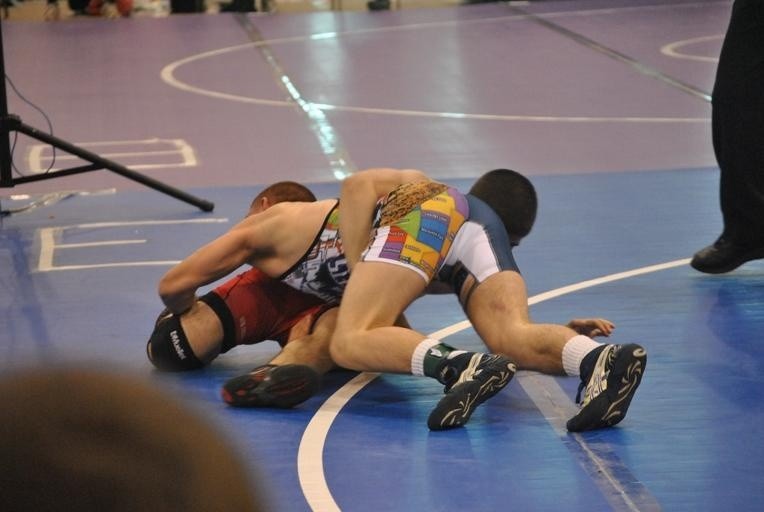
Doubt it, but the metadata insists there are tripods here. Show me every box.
[0,0,215,213]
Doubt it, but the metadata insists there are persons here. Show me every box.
[691,0,764,274]
[157,182,648,431]
[148,169,615,410]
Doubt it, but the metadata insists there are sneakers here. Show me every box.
[691,230,763,275]
[427,352,517,431]
[566,343,647,432]
[221,363,318,408]
